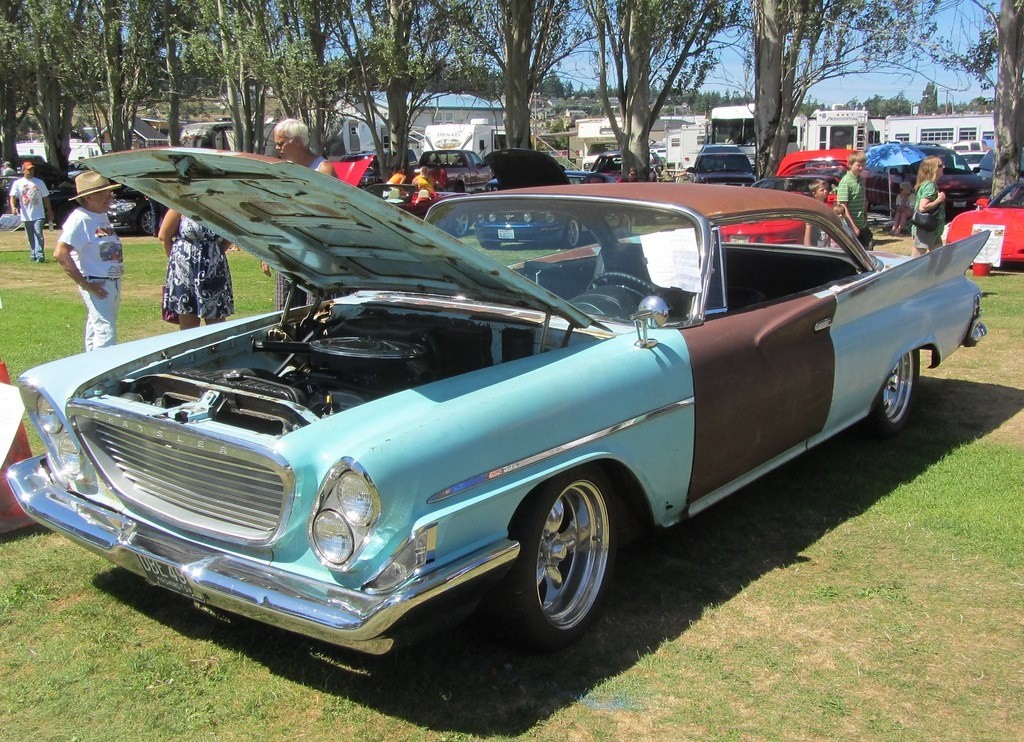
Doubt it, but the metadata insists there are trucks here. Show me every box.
[704,104,807,175]
[421,119,506,161]
[180,122,279,161]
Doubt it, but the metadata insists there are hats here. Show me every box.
[899,182,912,194]
[68,171,122,201]
[2,162,11,167]
[22,162,36,170]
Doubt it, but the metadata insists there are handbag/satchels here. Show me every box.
[909,207,941,231]
[160,285,179,325]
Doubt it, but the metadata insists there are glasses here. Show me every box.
[94,191,114,197]
[938,163,944,168]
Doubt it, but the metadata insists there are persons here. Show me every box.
[0,161,13,187]
[890,181,917,237]
[410,165,437,192]
[835,150,872,250]
[8,163,52,264]
[384,167,406,191]
[49,173,131,350]
[261,118,342,313]
[803,178,827,249]
[821,204,846,252]
[910,155,948,259]
[155,204,234,332]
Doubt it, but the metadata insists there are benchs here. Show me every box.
[525,242,857,322]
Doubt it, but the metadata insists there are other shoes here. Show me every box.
[30,254,45,263]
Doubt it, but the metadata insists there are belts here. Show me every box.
[83,275,120,281]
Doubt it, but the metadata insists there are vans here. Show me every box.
[340,148,418,191]
[360,184,474,237]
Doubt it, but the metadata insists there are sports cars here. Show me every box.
[945,182,1024,265]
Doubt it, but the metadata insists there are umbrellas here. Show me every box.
[862,141,929,237]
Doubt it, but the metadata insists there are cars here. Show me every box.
[686,152,756,188]
[472,148,632,249]
[866,145,993,223]
[951,139,1024,210]
[4,144,994,667]
[1,153,168,238]
[649,150,663,173]
[885,140,902,145]
[694,144,743,154]
[583,150,658,184]
[412,150,493,195]
[715,149,869,247]
